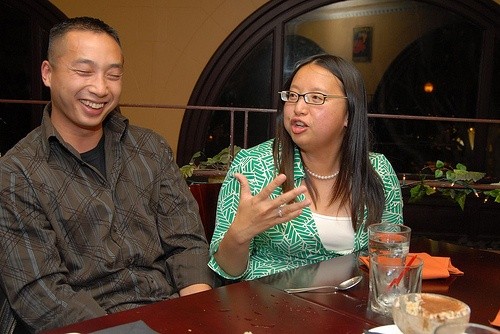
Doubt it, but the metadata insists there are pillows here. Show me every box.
[359,251,464,280]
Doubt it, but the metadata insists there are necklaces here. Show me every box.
[305,167,339,180]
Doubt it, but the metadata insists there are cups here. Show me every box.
[367,223,424,325]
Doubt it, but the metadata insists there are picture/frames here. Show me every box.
[352,24,373,62]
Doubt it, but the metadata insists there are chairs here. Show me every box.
[190,182,221,240]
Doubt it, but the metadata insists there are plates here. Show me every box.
[362,324,403,334]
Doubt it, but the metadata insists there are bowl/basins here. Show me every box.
[433,322,500,334]
[393,293,471,334]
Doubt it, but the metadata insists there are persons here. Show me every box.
[0,17,216,334]
[207,52,404,289]
[403,127,464,173]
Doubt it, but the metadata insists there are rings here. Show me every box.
[279,207,283,217]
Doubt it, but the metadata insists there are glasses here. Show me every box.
[277,91,348,106]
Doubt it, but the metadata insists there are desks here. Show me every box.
[36,279,376,334]
[256,236,500,331]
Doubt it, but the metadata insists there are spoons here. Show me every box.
[283,275,363,293]
